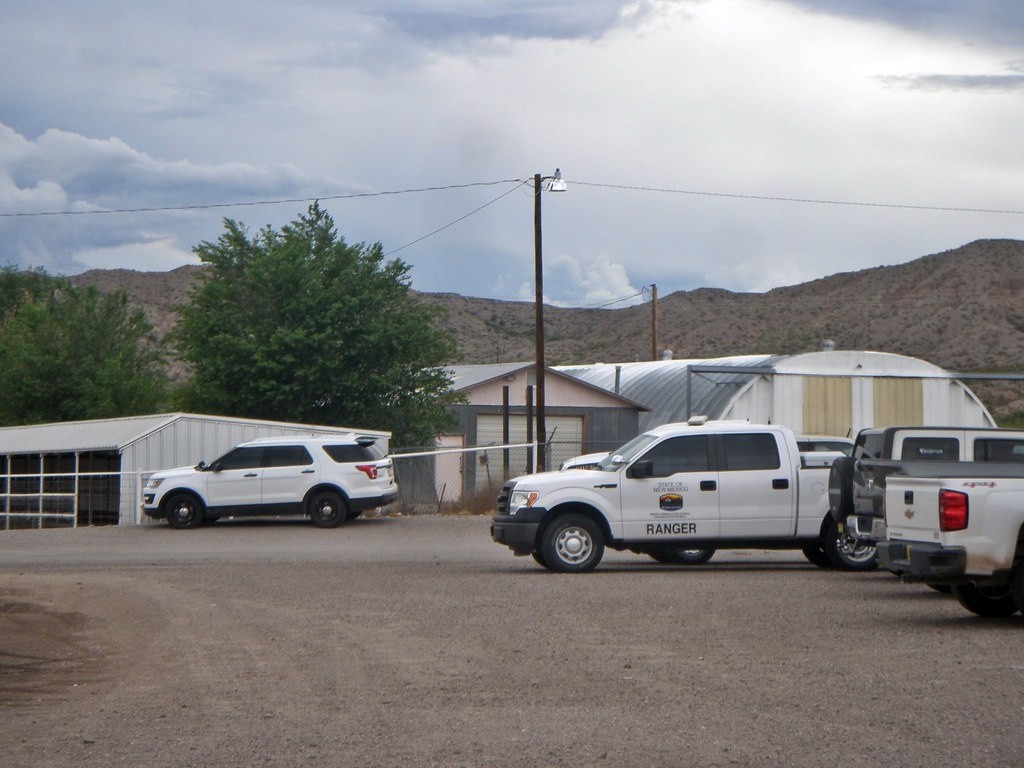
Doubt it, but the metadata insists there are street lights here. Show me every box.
[533,166,567,468]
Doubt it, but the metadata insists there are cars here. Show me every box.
[561,428,878,565]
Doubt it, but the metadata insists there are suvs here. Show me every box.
[839,423,1024,590]
[141,432,400,529]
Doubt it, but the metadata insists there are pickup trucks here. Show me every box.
[488,417,884,574]
[874,475,1024,623]
